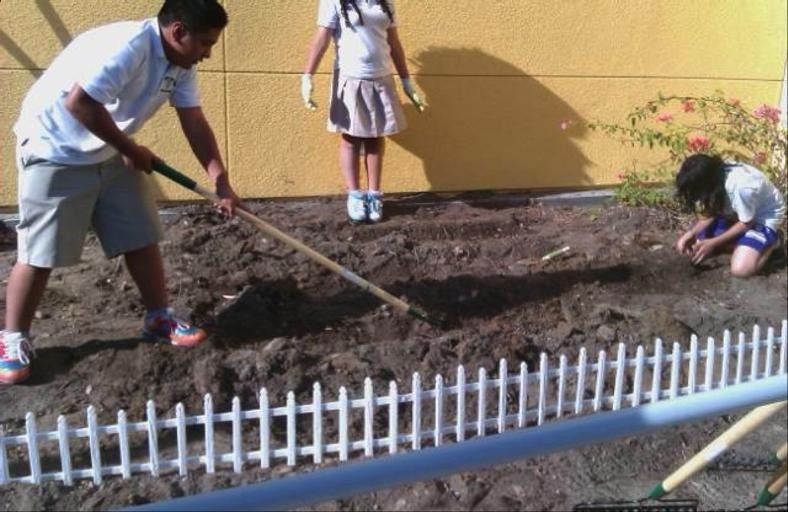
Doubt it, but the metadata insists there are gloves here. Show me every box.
[301,72,317,112]
[402,78,430,113]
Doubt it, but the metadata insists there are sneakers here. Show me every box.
[0,328,38,386]
[346,189,367,222]
[366,190,385,223]
[137,305,209,349]
[769,228,786,266]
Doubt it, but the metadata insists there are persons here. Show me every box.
[0,0,240,384]
[673,153,788,279]
[301,0,424,224]
